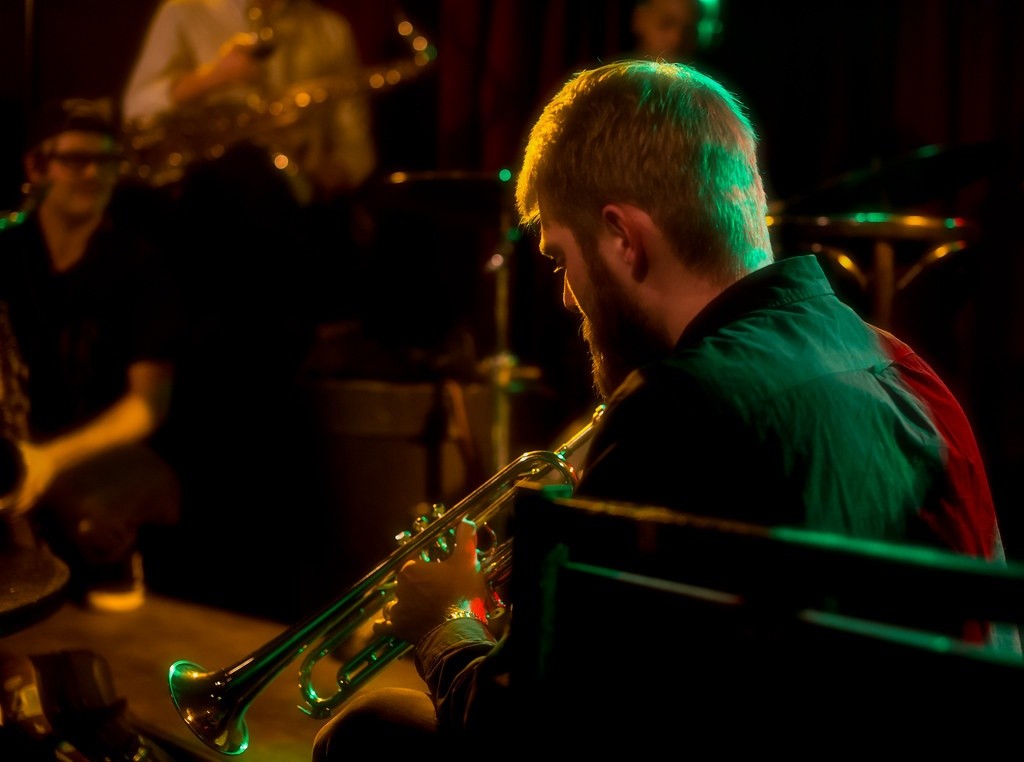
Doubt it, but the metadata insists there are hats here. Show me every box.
[26,97,119,144]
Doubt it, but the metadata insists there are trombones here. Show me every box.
[168,398,609,760]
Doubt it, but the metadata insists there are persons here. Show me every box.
[0,98,175,597]
[311,59,1023,762]
[117,3,376,616]
[628,1,723,65]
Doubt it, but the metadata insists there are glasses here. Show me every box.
[50,151,124,169]
